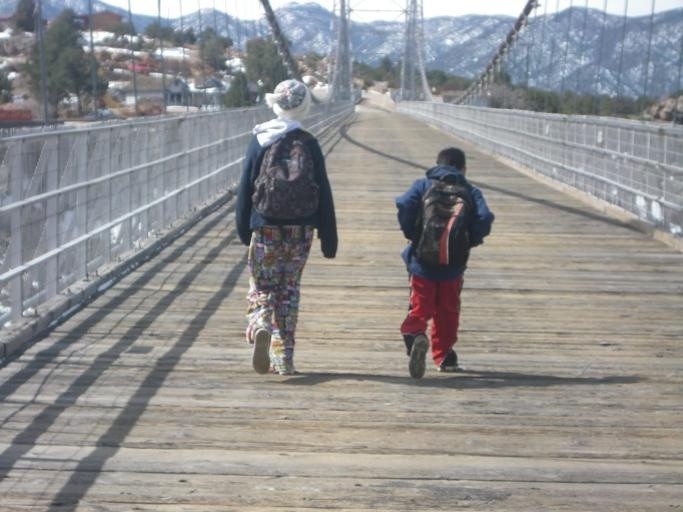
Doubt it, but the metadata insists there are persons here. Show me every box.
[396,148,495,380]
[235,80,338,376]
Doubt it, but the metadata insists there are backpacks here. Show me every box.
[414,178,475,273]
[252,131,320,220]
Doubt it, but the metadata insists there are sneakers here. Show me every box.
[252,329,271,374]
[437,351,458,372]
[273,363,295,375]
[408,334,427,378]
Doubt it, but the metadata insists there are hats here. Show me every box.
[264,78,311,121]
